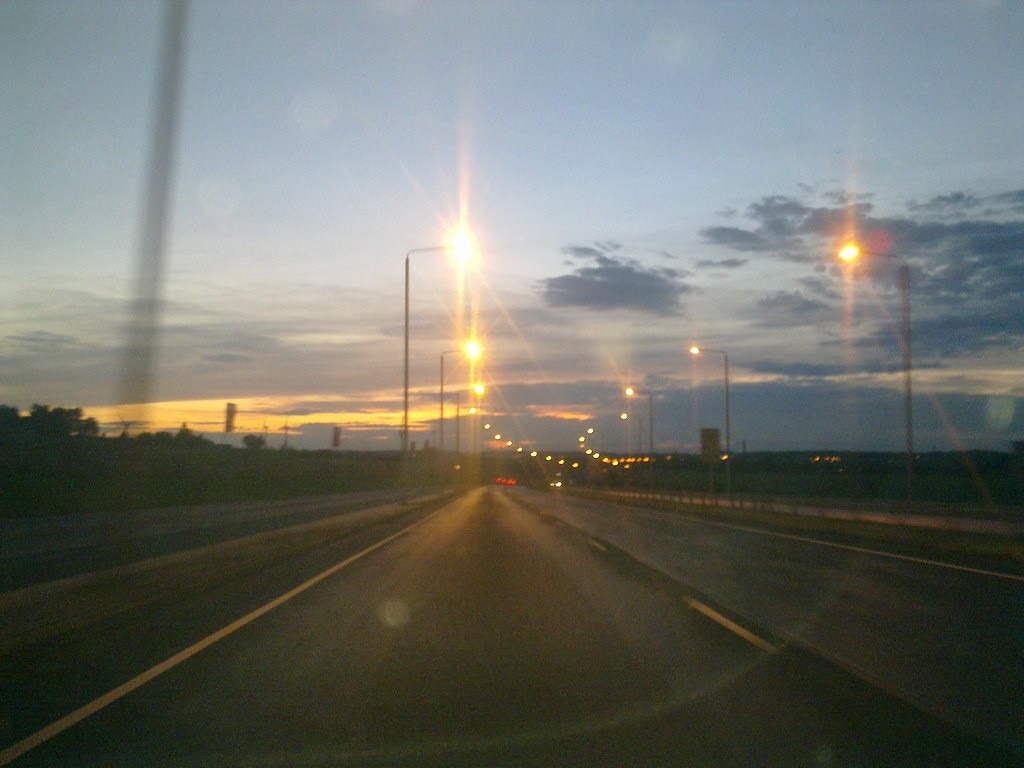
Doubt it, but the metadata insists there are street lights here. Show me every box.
[438,344,483,458]
[456,384,485,458]
[839,241,921,498]
[626,387,656,488]
[403,244,476,475]
[688,345,735,496]
